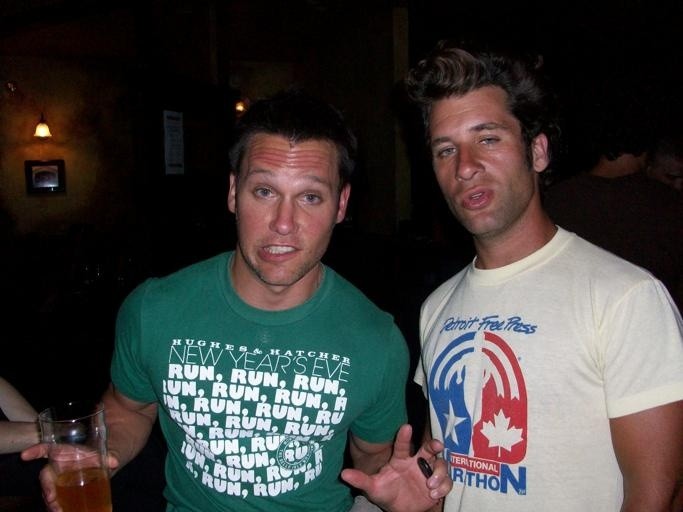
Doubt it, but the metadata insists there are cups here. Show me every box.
[38,401,111,512]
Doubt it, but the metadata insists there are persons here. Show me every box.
[0,377,41,454]
[21,91,453,512]
[405,48,682,512]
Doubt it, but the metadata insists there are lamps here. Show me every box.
[33,111,53,140]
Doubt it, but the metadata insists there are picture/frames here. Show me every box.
[24,158,66,196]
